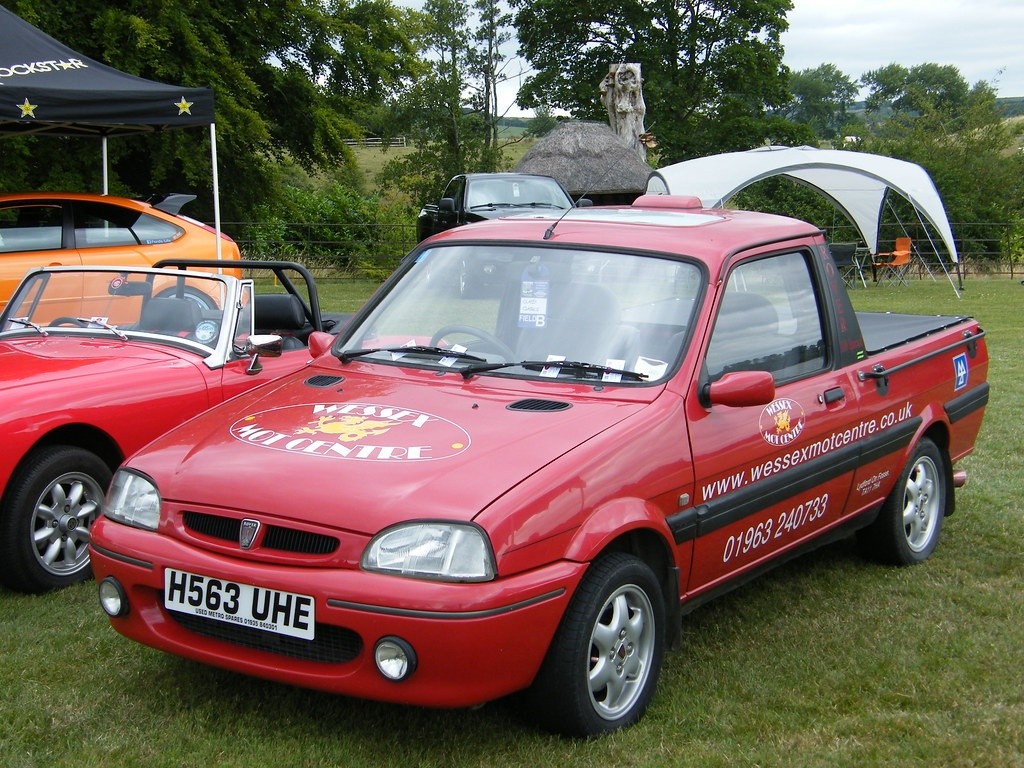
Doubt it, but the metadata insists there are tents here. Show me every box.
[0,6,226,313]
[644,146,965,292]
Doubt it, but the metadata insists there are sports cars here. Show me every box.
[0,257,448,595]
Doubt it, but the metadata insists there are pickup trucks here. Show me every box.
[88,190,992,743]
[417,172,593,296]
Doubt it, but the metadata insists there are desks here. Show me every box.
[856,248,870,288]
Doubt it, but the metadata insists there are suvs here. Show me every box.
[2,192,252,337]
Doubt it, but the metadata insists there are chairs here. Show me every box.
[873,237,911,288]
[236,294,309,355]
[828,244,857,290]
[141,296,203,338]
[703,290,778,370]
[515,281,617,364]
